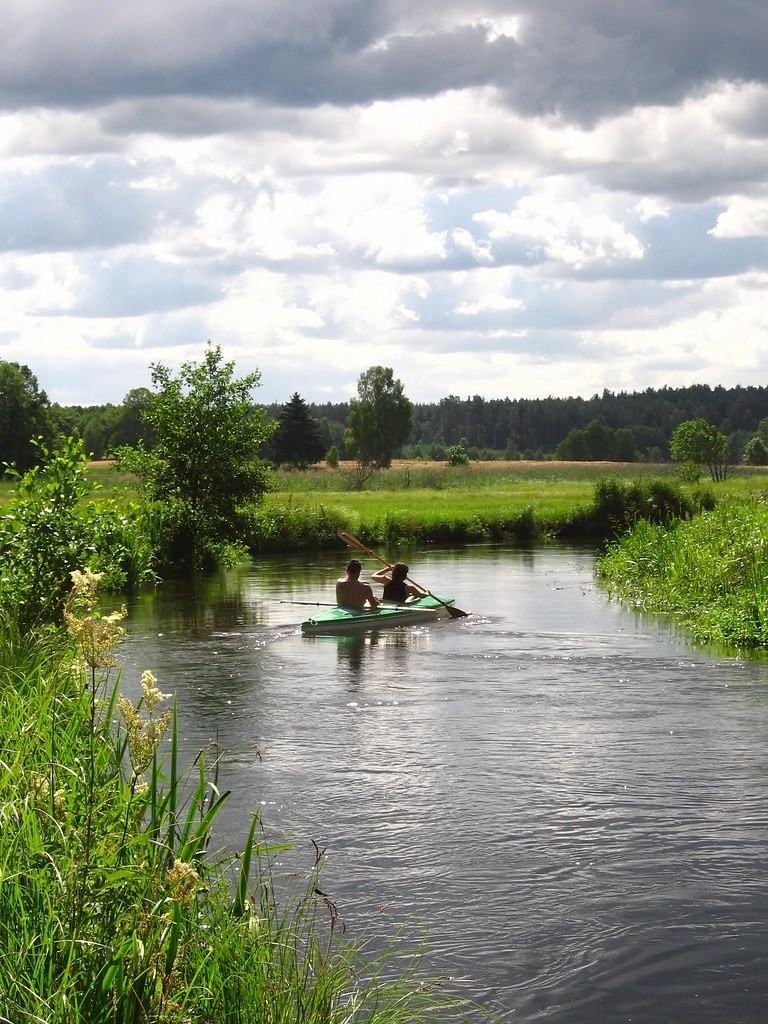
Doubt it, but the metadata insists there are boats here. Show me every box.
[299,595,456,637]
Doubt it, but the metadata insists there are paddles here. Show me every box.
[334,525,471,619]
[278,597,441,618]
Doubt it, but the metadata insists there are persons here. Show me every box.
[336,560,379,609]
[371,562,430,602]
